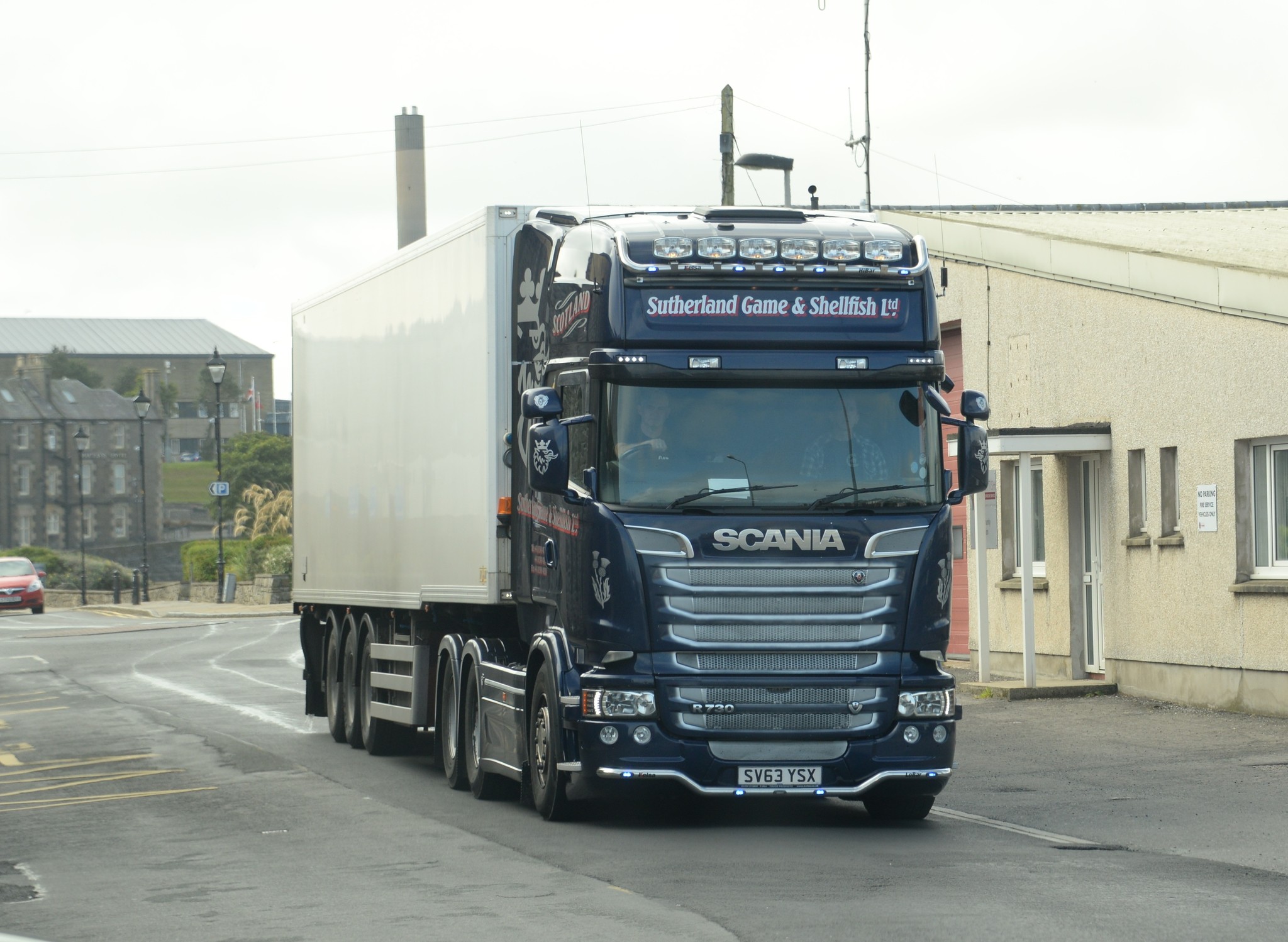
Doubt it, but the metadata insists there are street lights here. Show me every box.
[730,151,794,203]
[206,346,228,605]
[131,386,152,603]
[73,425,94,605]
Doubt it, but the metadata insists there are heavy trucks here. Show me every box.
[290,203,990,824]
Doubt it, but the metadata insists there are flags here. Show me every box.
[255,398,263,409]
[245,388,253,401]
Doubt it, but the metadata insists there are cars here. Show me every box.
[0,557,47,614]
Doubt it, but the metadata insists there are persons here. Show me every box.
[611,388,704,485]
[799,391,888,483]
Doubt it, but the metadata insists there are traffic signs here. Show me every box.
[207,482,229,497]
